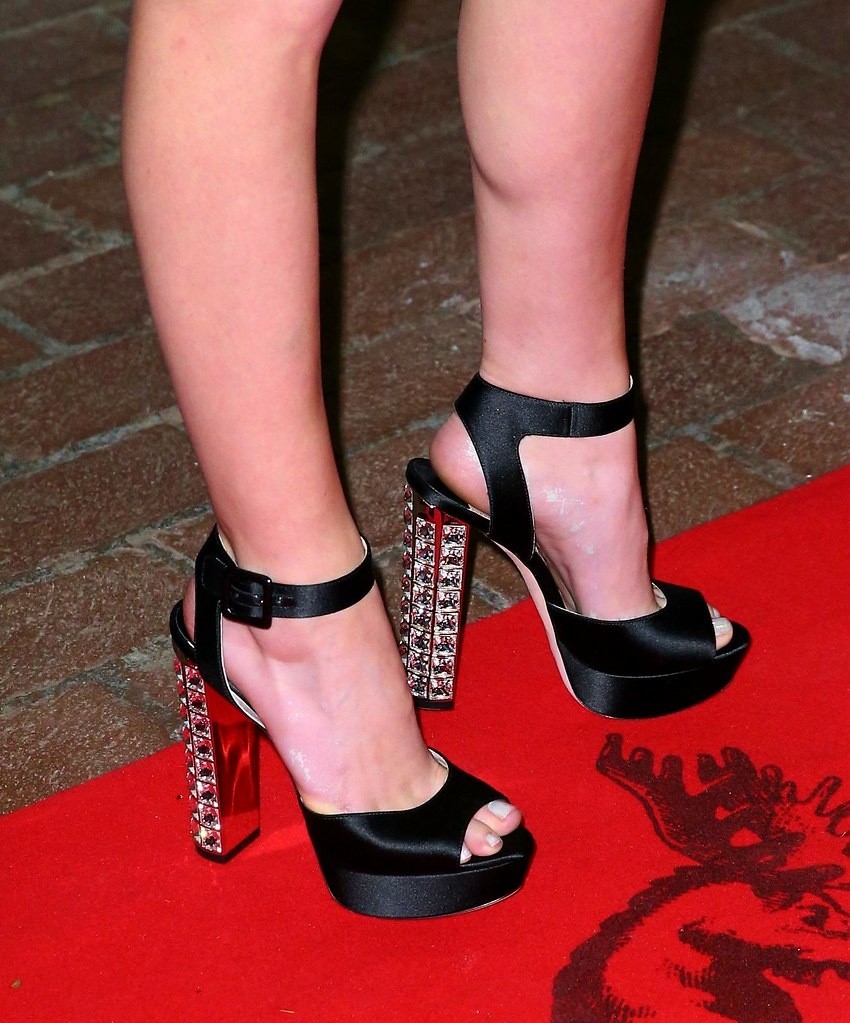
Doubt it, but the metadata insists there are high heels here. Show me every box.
[399,371,750,720]
[169,522,535,918]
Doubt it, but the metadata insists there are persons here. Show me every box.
[121,0,753,918]
